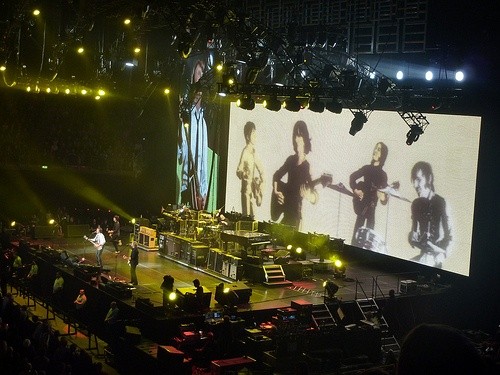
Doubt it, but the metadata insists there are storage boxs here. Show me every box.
[400,279,418,293]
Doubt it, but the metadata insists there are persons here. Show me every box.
[88,228,106,266]
[193,279,203,300]
[350,142,388,249]
[105,302,120,325]
[407,161,454,269]
[273,120,318,232]
[108,215,121,254]
[0,291,104,375]
[27,260,38,300]
[13,254,22,278]
[236,121,263,220]
[180,207,193,225]
[123,241,139,285]
[220,214,233,251]
[74,290,87,327]
[221,316,233,333]
[52,271,64,314]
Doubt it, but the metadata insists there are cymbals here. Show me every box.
[327,179,356,199]
[376,184,411,204]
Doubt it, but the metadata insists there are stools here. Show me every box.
[261,249,275,261]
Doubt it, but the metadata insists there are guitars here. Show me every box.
[350,180,400,215]
[123,254,134,267]
[105,228,113,238]
[83,235,103,250]
[270,173,333,221]
[249,174,264,208]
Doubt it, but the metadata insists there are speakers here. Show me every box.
[32,226,56,239]
[215,282,253,306]
[176,286,212,308]
[65,224,90,238]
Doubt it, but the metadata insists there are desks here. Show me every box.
[220,230,270,259]
[309,258,333,263]
[212,356,256,375]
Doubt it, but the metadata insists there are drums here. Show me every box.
[355,226,387,255]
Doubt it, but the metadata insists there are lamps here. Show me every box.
[286,245,307,260]
[0,10,464,145]
[322,281,339,300]
